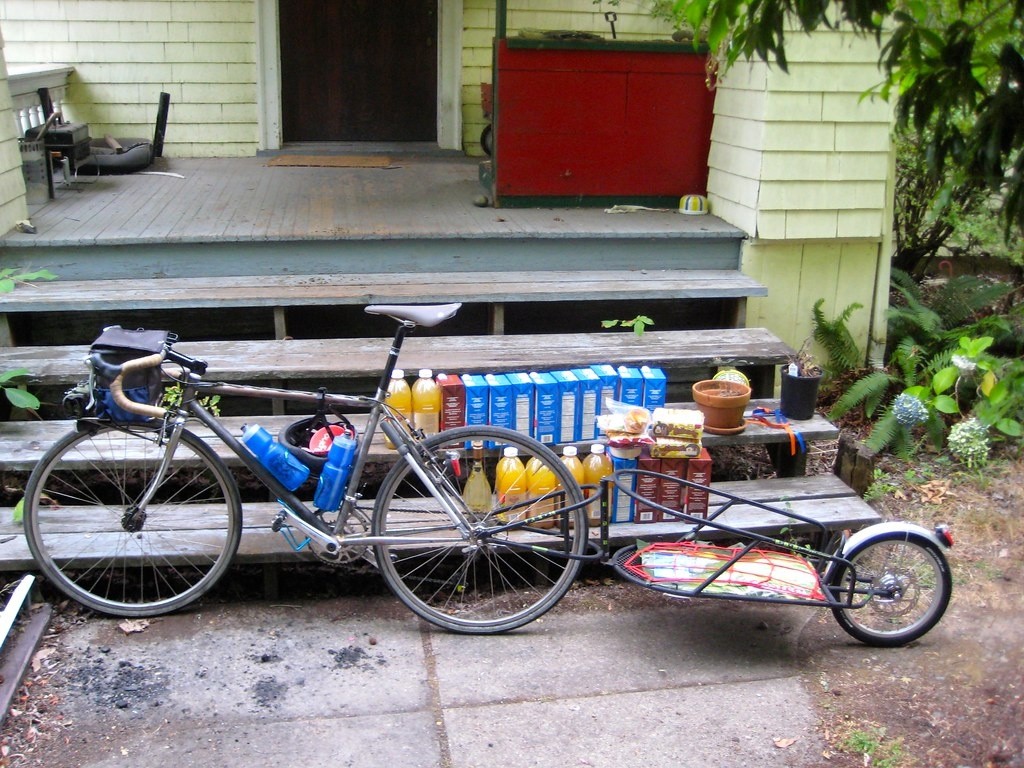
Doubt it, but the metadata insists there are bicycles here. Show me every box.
[22,303,588,637]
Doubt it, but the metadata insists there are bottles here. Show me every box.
[240,423,310,492]
[582,444,612,526]
[464,441,491,519]
[527,456,556,529]
[411,368,441,440]
[556,446,584,528]
[384,369,411,449]
[495,447,527,522]
[316,431,357,511]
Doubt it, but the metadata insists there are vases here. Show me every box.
[780,364,823,420]
[692,380,752,427]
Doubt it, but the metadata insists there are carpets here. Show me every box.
[264,154,392,169]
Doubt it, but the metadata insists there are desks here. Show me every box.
[491,34,716,210]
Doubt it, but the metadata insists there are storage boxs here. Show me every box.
[640,367,666,430]
[682,448,712,523]
[591,365,620,436]
[658,457,683,521]
[617,367,642,408]
[633,447,658,524]
[529,372,557,446]
[435,373,464,448]
[506,373,534,438]
[88,325,167,421]
[571,369,600,441]
[461,374,487,449]
[606,447,637,523]
[484,374,509,449]
[550,370,578,443]
